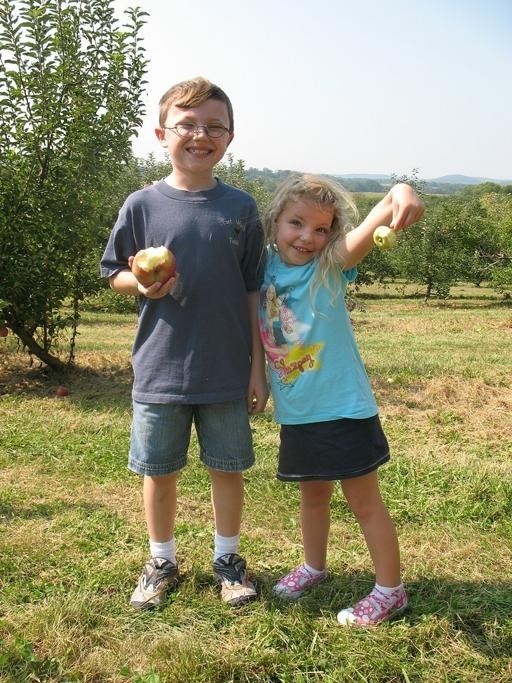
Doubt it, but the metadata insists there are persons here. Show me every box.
[100,75,270,611]
[256,172,425,629]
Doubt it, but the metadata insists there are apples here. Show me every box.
[373,226,397,248]
[57,385,69,396]
[0,327,8,337]
[131,246,177,288]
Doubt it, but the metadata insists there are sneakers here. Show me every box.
[272,565,322,601]
[334,587,407,626]
[210,554,256,603]
[126,558,177,604]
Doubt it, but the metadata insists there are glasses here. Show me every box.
[163,117,234,139]
